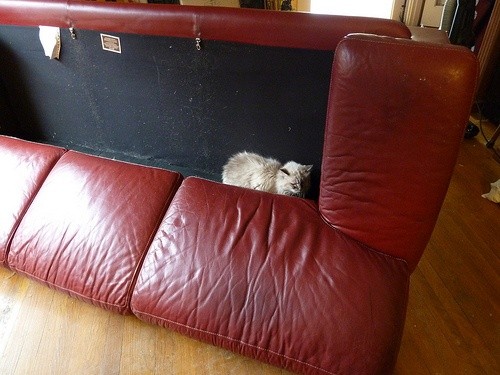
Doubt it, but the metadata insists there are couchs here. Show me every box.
[0,0,480,374]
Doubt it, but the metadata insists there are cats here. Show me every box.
[222,150,314,198]
